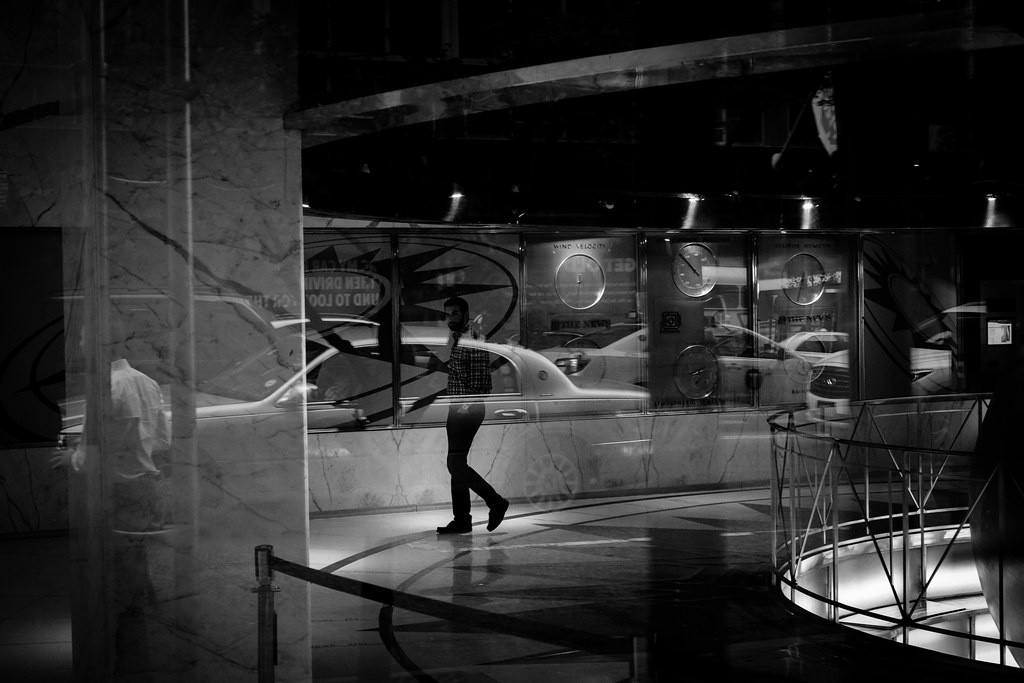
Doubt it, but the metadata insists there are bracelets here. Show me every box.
[463,404,468,410]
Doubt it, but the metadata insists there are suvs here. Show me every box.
[267,311,381,332]
[64,289,276,416]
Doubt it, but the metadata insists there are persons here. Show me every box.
[427,297,509,534]
[45,308,171,621]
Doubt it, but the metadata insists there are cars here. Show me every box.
[58,326,650,513]
[502,330,583,362]
[804,301,987,433]
[777,328,853,361]
[541,318,811,408]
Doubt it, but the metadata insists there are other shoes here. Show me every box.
[487,499,509,531]
[437,521,472,534]
